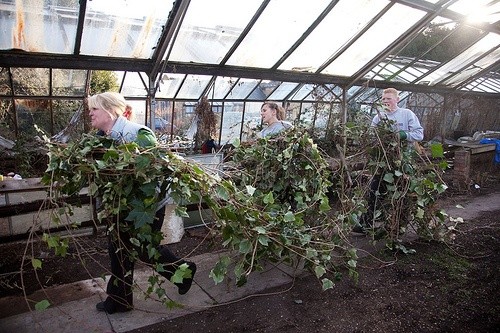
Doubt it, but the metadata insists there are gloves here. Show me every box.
[384,130,407,146]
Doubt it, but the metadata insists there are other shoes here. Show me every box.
[179,261,197,294]
[350,224,373,233]
[96,301,134,313]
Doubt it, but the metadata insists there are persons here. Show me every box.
[243,102,314,271]
[350,87,423,236]
[87,91,196,313]
